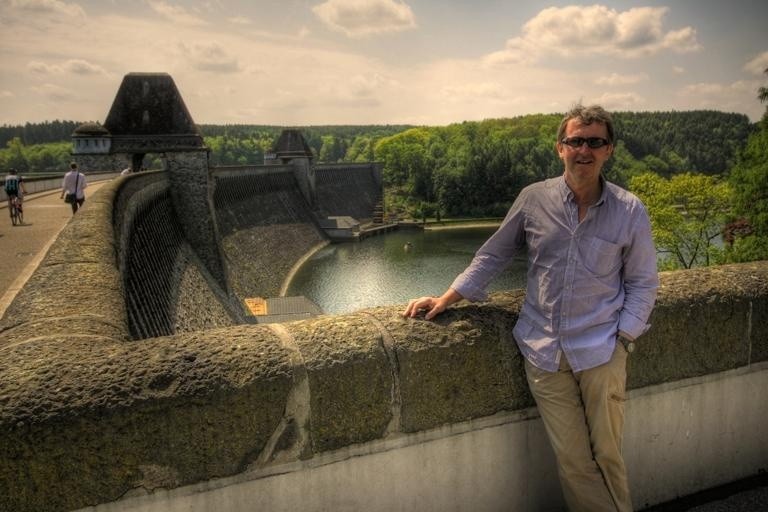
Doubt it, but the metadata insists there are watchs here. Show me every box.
[616,334,636,354]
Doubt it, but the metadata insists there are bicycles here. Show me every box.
[7,189,27,226]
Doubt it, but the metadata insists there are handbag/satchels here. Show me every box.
[65,193,77,204]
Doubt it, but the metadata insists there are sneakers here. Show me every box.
[16,204,23,213]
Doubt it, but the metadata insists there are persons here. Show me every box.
[398,105,659,512]
[60,162,88,215]
[120,168,132,174]
[4,168,27,217]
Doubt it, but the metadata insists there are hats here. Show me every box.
[71,162,77,169]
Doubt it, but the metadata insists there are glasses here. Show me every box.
[561,136,610,149]
